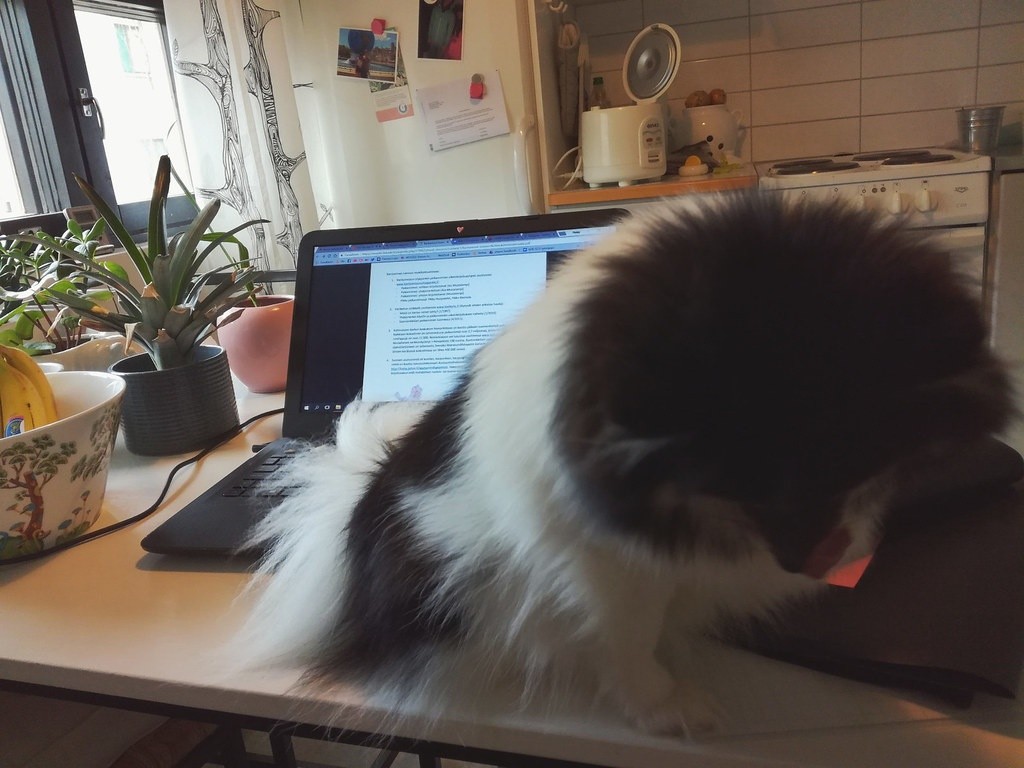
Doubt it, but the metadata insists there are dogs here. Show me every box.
[205,177,1022,753]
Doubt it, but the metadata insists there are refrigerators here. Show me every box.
[276,0,577,232]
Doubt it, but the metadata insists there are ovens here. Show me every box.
[900,228,987,306]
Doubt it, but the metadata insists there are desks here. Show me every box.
[0,366,1024,767]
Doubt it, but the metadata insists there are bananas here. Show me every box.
[0,345,59,439]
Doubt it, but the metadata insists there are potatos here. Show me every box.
[684,87,725,107]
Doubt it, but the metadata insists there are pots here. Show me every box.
[553,22,681,190]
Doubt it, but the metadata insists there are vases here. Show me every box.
[0,371,127,560]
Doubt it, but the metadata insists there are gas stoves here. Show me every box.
[753,145,992,227]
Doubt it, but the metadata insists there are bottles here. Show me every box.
[667,103,743,164]
[587,76,611,110]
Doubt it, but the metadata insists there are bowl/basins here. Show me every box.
[0,371,127,562]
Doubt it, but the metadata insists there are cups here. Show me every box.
[954,104,1008,151]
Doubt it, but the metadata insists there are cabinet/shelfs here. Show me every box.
[549,155,757,213]
[985,155,1024,365]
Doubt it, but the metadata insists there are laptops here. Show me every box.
[141,208,633,554]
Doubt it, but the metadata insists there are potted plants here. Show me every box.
[0,154,296,456]
